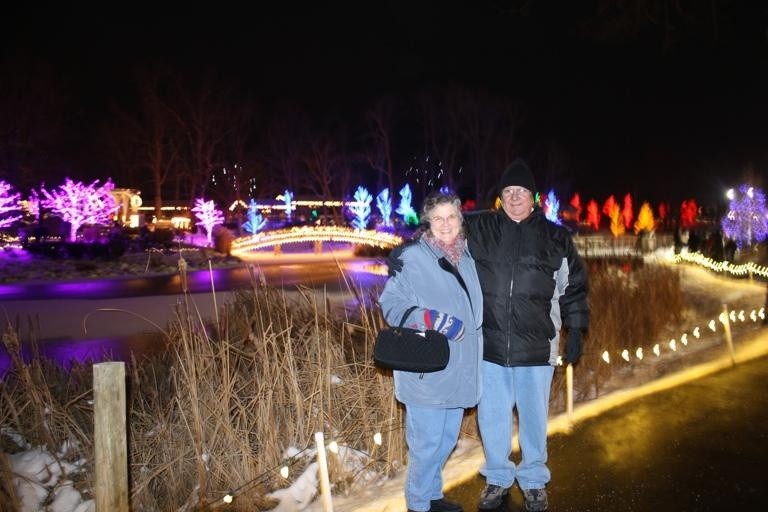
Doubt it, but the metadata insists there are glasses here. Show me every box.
[502,188,530,197]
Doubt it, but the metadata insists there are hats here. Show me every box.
[498,157,536,200]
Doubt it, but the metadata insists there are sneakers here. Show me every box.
[521,487,549,512]
[430,497,464,512]
[478,484,509,511]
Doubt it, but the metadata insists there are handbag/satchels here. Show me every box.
[374,326,450,373]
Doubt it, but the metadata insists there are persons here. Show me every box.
[386,161,591,511]
[378,191,483,512]
[634,221,737,265]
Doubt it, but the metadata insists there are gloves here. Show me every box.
[424,309,465,343]
[384,245,404,277]
[562,328,582,366]
